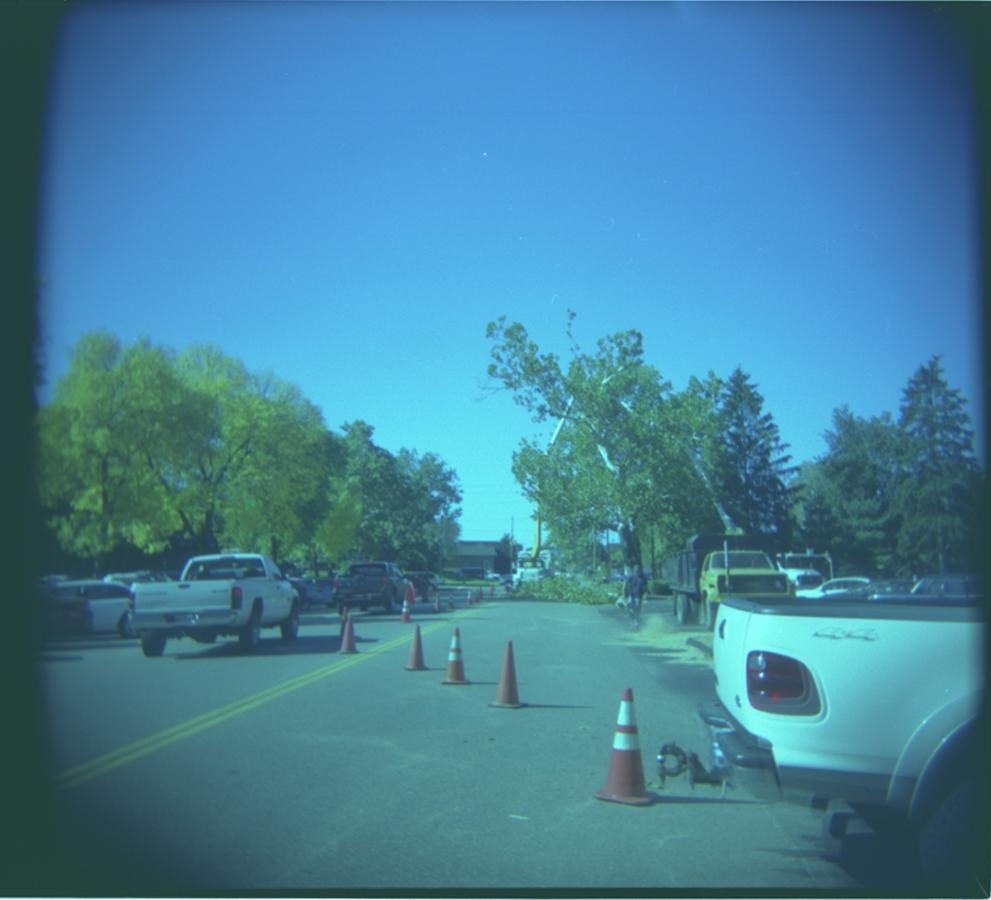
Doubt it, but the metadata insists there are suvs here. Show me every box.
[104,569,173,590]
[406,571,439,601]
[54,580,140,640]
[512,559,546,586]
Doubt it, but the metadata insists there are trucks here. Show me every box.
[669,533,832,632]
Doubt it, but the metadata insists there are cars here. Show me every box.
[286,576,334,609]
[852,572,973,598]
[457,568,500,581]
[794,576,871,598]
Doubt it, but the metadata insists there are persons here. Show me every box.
[620,560,649,631]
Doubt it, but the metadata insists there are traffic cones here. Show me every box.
[432,592,455,612]
[465,585,493,606]
[593,688,659,806]
[439,627,473,684]
[486,641,524,708]
[336,605,357,654]
[405,625,429,671]
[399,599,411,624]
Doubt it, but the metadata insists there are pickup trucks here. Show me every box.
[130,552,300,658]
[333,560,409,611]
[657,588,987,889]
